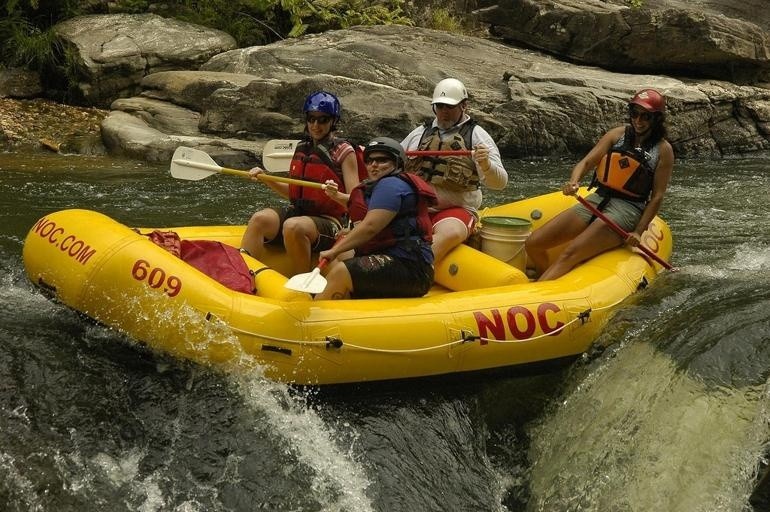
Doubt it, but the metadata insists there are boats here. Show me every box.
[22,186,674,386]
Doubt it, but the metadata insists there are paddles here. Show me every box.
[263,138,475,173]
[285,236,342,293]
[170,146,326,190]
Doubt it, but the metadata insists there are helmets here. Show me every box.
[363,137,405,171]
[302,91,341,117]
[431,78,469,107]
[630,89,665,113]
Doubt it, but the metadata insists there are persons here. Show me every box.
[239,90,362,274]
[314,137,436,300]
[522,88,677,284]
[399,78,507,270]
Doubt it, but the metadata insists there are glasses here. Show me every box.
[364,157,394,164]
[435,101,464,109]
[307,116,333,124]
[630,109,653,121]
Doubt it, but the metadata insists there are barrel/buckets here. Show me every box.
[481,214,532,274]
